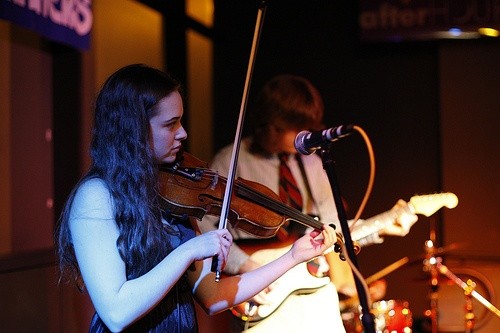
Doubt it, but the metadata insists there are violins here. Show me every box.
[154,150,361,262]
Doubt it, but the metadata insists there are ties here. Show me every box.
[278,154,303,212]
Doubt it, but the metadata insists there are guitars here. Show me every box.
[221,192,459,322]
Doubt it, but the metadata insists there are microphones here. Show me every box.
[293,124,356,155]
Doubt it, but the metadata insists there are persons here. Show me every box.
[196,73,418,333]
[58,63,337,333]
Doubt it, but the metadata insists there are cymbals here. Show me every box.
[409,243,460,261]
[408,278,456,286]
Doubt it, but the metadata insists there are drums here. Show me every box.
[342,298,412,333]
